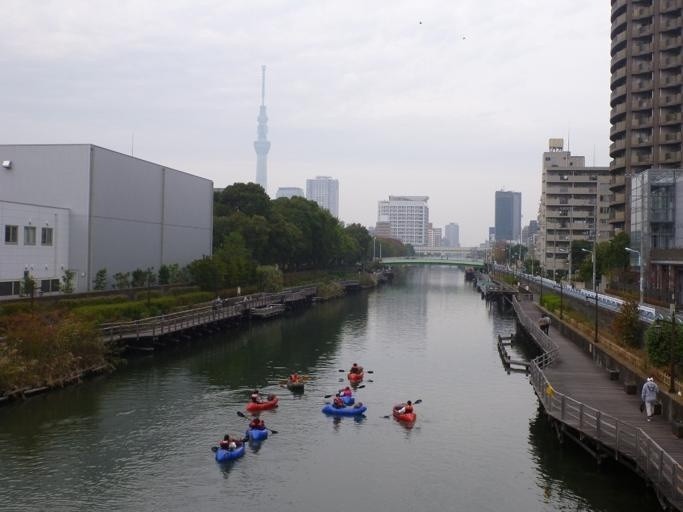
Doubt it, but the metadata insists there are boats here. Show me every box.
[320,402,367,417]
[463,265,500,301]
[339,396,354,406]
[211,438,246,463]
[244,394,279,411]
[245,427,269,443]
[285,376,304,392]
[391,404,416,425]
[347,372,363,383]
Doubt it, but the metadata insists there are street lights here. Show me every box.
[557,247,573,288]
[623,247,644,306]
[580,247,595,292]
[373,236,377,260]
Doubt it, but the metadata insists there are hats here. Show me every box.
[647,377,654,382]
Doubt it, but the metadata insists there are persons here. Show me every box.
[544,315,551,336]
[338,386,351,397]
[220,434,238,450]
[294,371,299,382]
[641,377,660,422]
[290,372,295,381]
[350,364,361,374]
[394,401,413,414]
[540,315,546,333]
[571,279,575,290]
[334,394,346,409]
[236,286,241,297]
[249,418,264,430]
[251,390,264,404]
[216,295,223,304]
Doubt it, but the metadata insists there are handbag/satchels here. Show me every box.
[654,403,661,416]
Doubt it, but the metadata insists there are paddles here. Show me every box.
[339,369,373,374]
[324,394,336,398]
[384,400,422,418]
[237,411,277,434]
[338,379,373,382]
